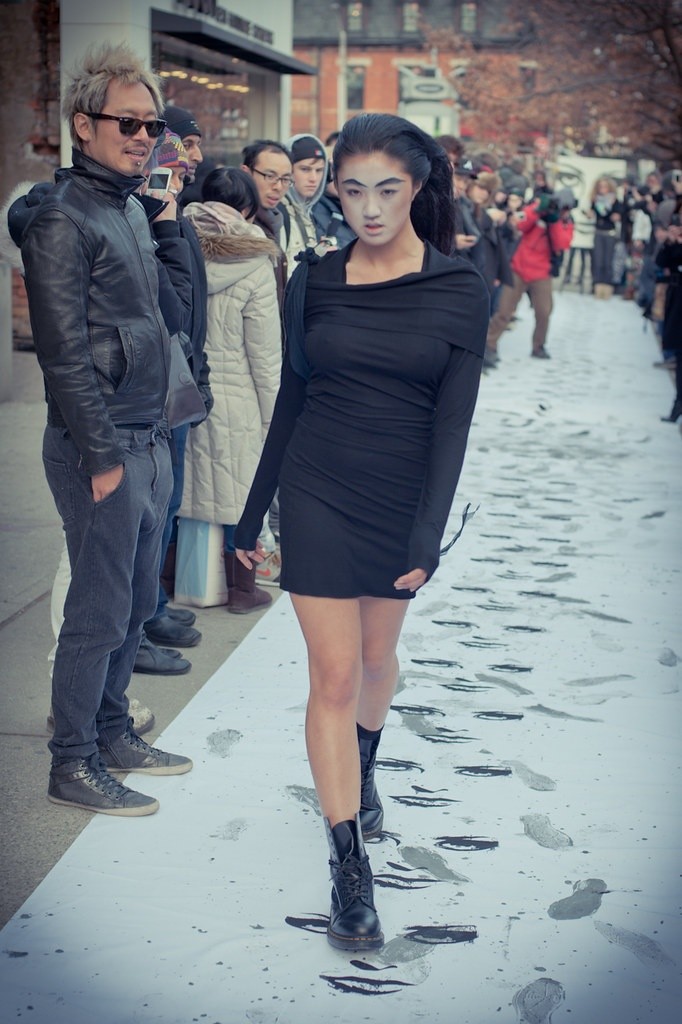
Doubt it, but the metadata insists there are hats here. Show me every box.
[509,187,524,197]
[291,137,325,164]
[154,126,190,173]
[165,106,203,142]
[454,157,482,182]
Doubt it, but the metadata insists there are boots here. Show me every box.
[225,548,272,614]
[350,722,383,834]
[321,812,384,951]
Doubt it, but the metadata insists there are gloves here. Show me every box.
[536,196,561,224]
[190,385,214,429]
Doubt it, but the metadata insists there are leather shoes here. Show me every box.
[133,636,192,676]
[151,604,204,649]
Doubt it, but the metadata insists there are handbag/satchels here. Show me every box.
[168,332,207,431]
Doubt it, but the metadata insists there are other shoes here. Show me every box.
[653,360,677,370]
[661,399,682,422]
[485,346,499,362]
[273,530,281,547]
[532,345,551,359]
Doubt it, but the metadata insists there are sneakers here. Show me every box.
[251,549,282,589]
[45,757,159,819]
[98,719,193,777]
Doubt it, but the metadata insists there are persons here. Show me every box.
[13,57,573,814]
[235,116,490,950]
[563,157,682,423]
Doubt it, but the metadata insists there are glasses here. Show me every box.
[82,111,168,139]
[251,166,296,189]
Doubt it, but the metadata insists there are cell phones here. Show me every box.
[146,167,172,200]
